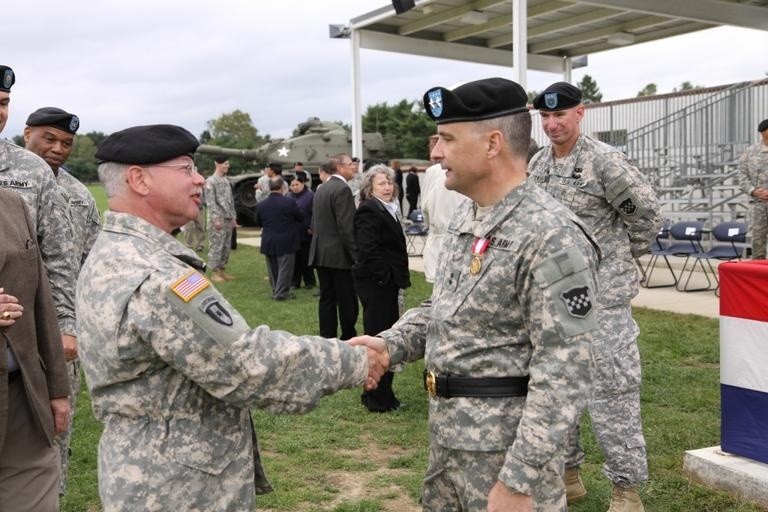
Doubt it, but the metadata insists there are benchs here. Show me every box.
[650,153,749,233]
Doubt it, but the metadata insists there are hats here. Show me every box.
[423,78,530,124]
[269,163,282,174]
[758,119,768,131]
[216,157,227,164]
[289,171,309,182]
[0,65,200,167]
[534,82,581,110]
[352,158,360,162]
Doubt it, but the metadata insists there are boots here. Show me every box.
[563,466,588,504]
[607,484,645,512]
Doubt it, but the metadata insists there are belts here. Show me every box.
[423,362,529,399]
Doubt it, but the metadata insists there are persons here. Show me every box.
[0,180,76,512]
[185,166,205,251]
[22,103,99,279]
[735,116,768,260]
[203,154,239,285]
[524,80,664,512]
[0,59,79,498]
[252,150,470,303]
[75,122,390,512]
[349,161,412,416]
[344,76,598,511]
[305,151,357,342]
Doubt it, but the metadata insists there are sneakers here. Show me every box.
[187,242,408,411]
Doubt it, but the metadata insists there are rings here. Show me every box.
[4,311,10,319]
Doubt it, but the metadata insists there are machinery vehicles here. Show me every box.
[195,115,389,228]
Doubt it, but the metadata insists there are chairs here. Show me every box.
[403,209,427,256]
[655,221,713,291]
[691,220,749,297]
[640,220,677,288]
